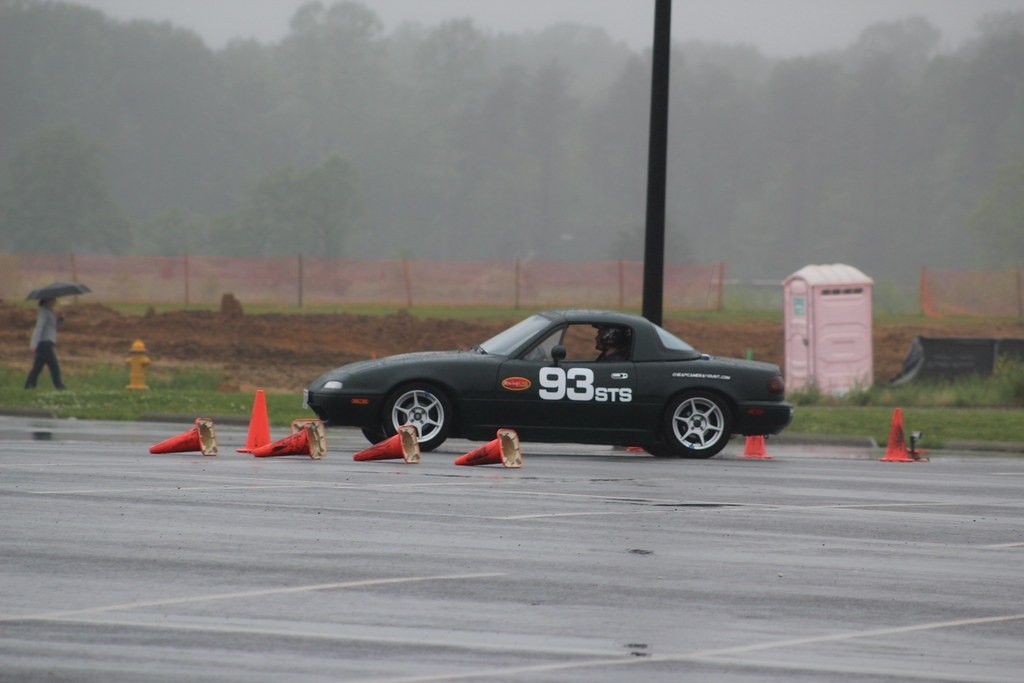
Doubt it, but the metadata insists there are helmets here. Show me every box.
[592,324,627,350]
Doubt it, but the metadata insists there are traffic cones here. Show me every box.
[627,447,643,452]
[738,433,774,460]
[148,416,219,456]
[877,407,916,462]
[250,422,326,459]
[235,389,274,454]
[455,428,523,469]
[352,425,421,464]
[291,418,326,455]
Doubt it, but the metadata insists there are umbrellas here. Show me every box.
[24,282,92,301]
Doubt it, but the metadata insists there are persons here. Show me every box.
[24,296,68,389]
[594,324,629,361]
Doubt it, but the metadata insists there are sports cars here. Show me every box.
[303,309,793,459]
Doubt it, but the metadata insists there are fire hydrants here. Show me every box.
[125,338,151,390]
